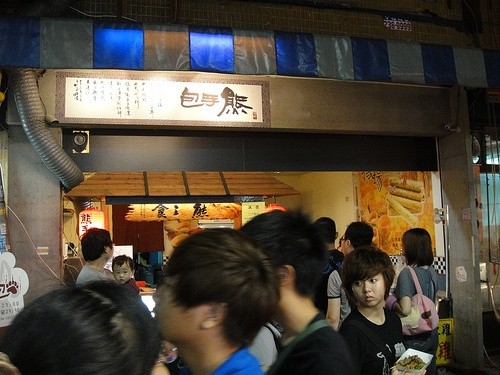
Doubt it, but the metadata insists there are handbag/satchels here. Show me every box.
[396,265,440,334]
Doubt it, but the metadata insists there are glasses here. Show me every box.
[157,271,182,289]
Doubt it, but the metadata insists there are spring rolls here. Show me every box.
[386,177,425,230]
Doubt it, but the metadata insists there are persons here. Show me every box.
[387,228,440,375]
[326,222,374,331]
[340,245,427,375]
[0,207,356,375]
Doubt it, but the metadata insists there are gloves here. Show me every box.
[386,295,397,311]
